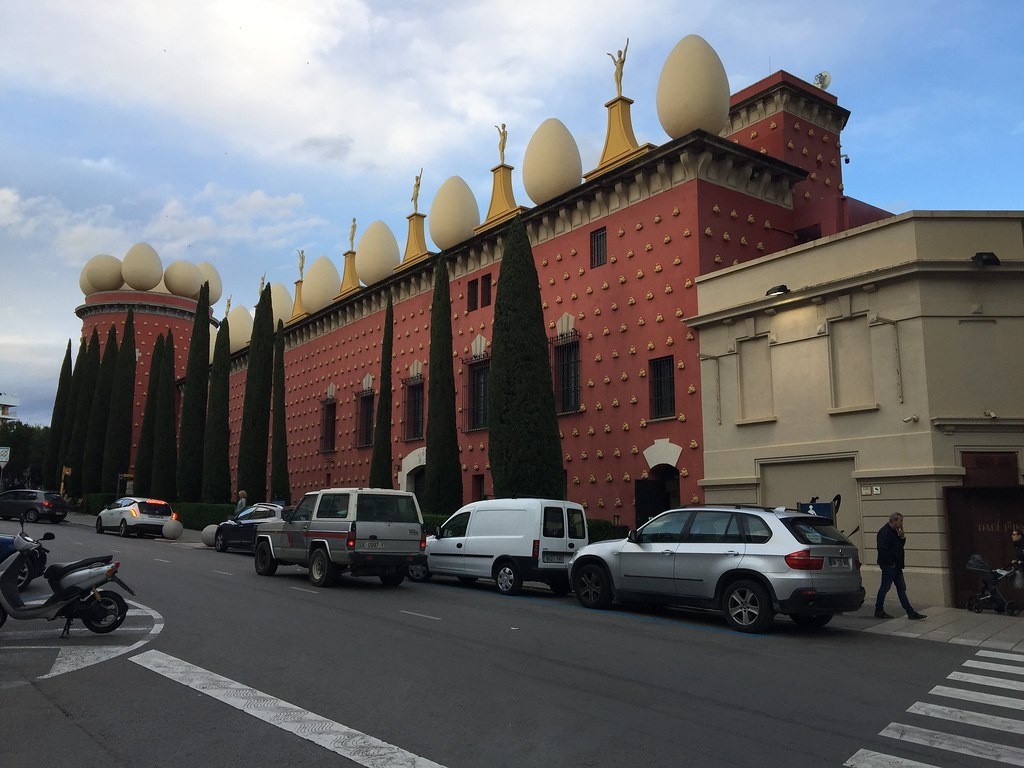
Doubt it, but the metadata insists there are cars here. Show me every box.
[95,497,177,537]
[0,488,71,523]
[0,532,47,592]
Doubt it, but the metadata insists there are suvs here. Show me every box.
[215,503,312,553]
[567,503,867,633]
[253,487,427,588]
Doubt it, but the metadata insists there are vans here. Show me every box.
[407,498,589,595]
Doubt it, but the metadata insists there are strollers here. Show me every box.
[964,554,1022,616]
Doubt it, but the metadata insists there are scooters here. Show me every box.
[0,513,137,639]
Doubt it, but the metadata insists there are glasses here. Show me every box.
[1011,533,1015,536]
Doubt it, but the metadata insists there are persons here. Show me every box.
[234,490,247,517]
[1011,529,1024,610]
[874,512,927,620]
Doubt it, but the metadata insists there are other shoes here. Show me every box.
[908,610,928,620]
[874,607,894,619]
